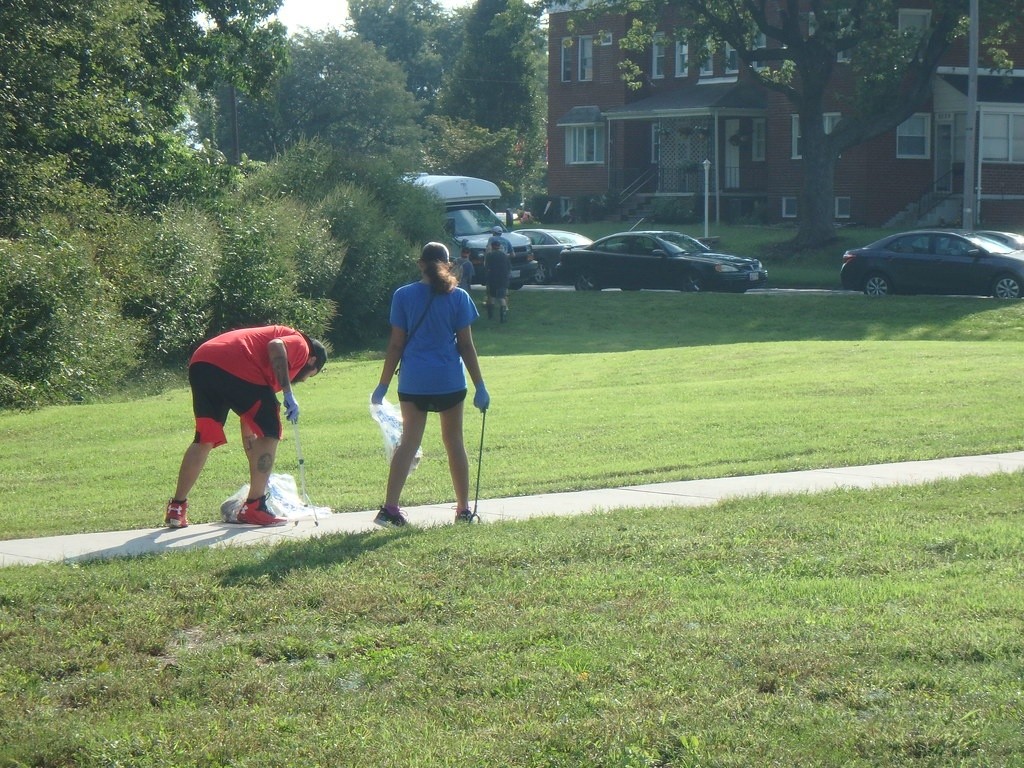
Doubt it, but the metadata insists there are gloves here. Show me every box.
[371,384,388,404]
[472,381,490,413]
[283,392,299,424]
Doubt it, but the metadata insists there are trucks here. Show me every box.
[400,173,539,291]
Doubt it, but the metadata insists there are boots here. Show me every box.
[500,306,507,323]
[487,304,495,320]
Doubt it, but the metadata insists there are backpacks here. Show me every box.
[448,257,470,284]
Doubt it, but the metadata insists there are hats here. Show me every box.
[462,246,471,253]
[493,226,503,234]
[298,328,328,371]
[490,240,500,245]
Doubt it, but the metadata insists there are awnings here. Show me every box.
[555,104,604,126]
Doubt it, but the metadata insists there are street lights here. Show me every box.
[702,158,712,238]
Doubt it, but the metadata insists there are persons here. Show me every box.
[371,243,490,529]
[480,241,513,323]
[449,247,476,294]
[165,326,327,529]
[484,226,515,311]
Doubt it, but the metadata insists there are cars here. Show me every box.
[555,231,768,290]
[509,228,593,285]
[839,231,1024,299]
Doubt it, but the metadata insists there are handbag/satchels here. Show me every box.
[369,395,424,473]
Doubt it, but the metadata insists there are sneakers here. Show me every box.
[236,492,289,527]
[163,497,189,528]
[455,510,473,523]
[373,505,409,530]
